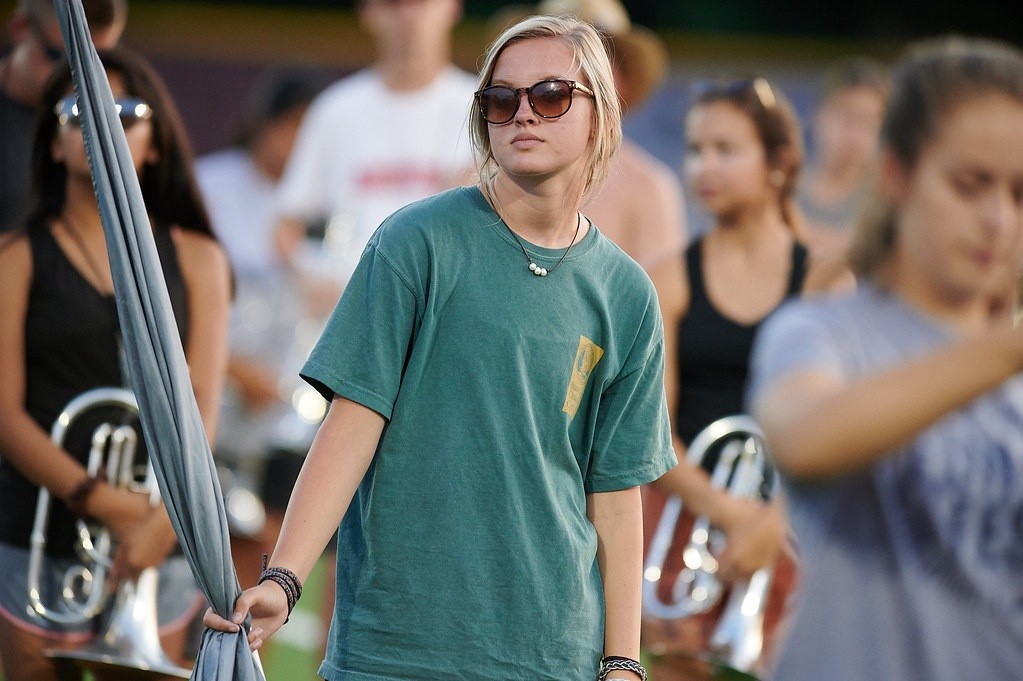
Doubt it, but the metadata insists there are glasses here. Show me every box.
[21,11,70,70]
[696,74,774,114]
[54,96,153,131]
[473,79,595,125]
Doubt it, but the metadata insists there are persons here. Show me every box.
[202,16,678,681]
[193,76,325,533]
[258,0,496,664]
[640,46,1023,681]
[546,0,683,272]
[0,1,239,681]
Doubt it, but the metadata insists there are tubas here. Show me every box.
[637,415,792,672]
[26,381,197,680]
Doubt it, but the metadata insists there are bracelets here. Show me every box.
[258,554,302,625]
[598,656,648,681]
[69,468,108,513]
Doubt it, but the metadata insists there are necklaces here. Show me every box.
[486,173,580,276]
